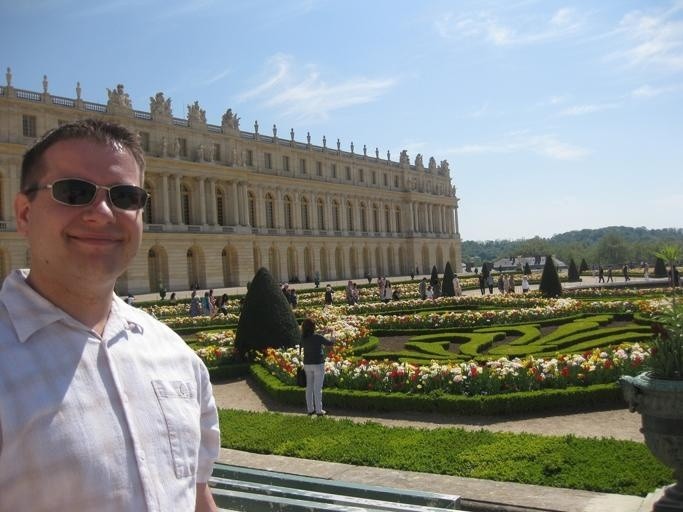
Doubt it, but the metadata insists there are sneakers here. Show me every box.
[310,409,328,416]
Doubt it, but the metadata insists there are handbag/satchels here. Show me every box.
[297,367,306,387]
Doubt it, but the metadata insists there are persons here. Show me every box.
[599,268,606,283]
[314,271,320,281]
[299,317,335,418]
[644,263,650,278]
[623,265,631,281]
[124,293,136,306]
[169,292,178,303]
[189,282,297,320]
[147,305,158,320]
[452,274,463,296]
[0,116,224,512]
[324,273,401,306]
[669,264,680,286]
[419,278,427,300]
[425,286,434,299]
[606,267,614,282]
[479,272,516,294]
[522,275,531,292]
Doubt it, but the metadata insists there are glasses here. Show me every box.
[25,177,151,212]
